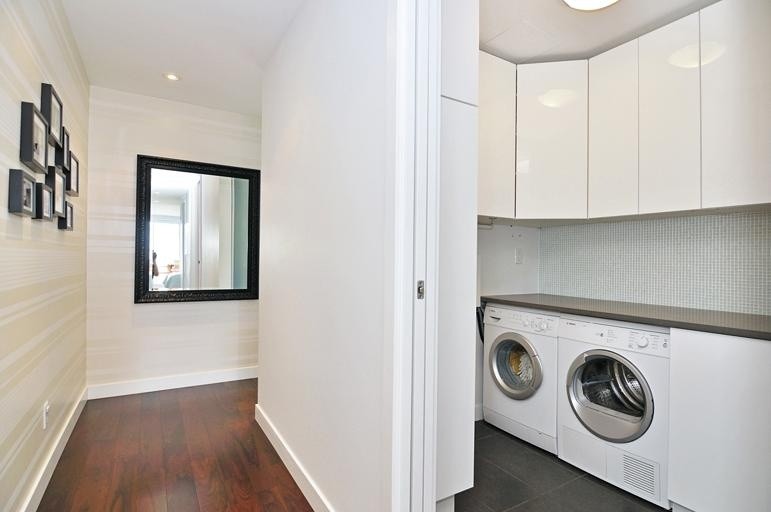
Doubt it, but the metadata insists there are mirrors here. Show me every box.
[134,153,261,305]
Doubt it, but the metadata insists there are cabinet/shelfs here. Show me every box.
[703,0,770,210]
[640,8,704,215]
[436,94,479,505]
[437,0,480,106]
[665,321,770,511]
[587,36,640,220]
[517,58,587,222]
[480,48,517,224]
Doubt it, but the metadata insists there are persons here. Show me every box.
[152,249,159,279]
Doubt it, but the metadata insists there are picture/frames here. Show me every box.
[10,81,81,232]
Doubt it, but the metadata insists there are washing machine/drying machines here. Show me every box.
[482,302,559,458]
[558,313,671,512]
